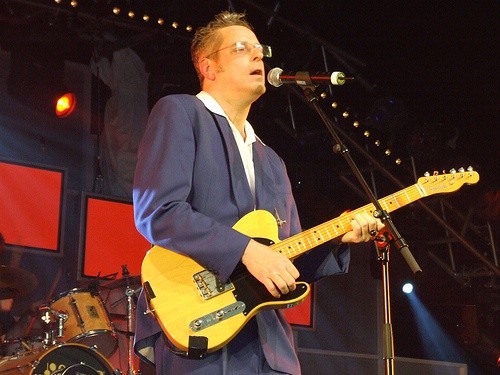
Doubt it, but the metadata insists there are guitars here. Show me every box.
[140,166,480,359]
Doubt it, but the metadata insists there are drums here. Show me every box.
[46,287,118,359]
[1,334,46,356]
[0,345,118,375]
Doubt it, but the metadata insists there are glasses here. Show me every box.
[207,44,272,59]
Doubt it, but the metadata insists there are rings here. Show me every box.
[369,230,376,236]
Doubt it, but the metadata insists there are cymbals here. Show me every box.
[101,276,139,288]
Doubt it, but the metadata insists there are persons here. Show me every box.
[132,12,386,375]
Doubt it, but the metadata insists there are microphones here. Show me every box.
[267,68,346,87]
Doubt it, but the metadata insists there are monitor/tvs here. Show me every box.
[76,192,155,285]
[0,157,69,256]
